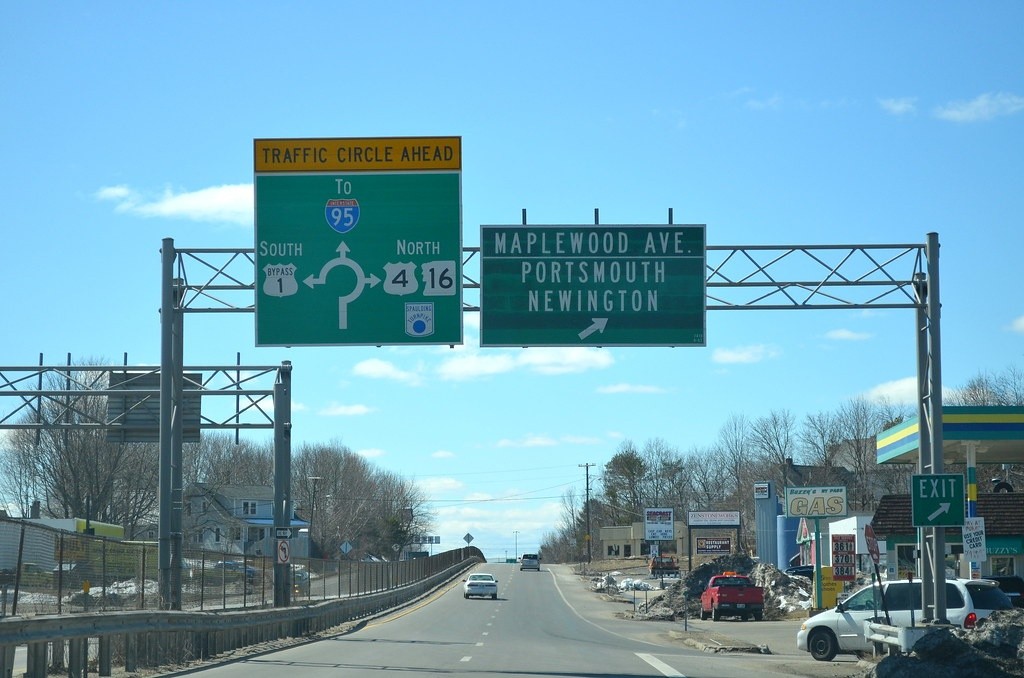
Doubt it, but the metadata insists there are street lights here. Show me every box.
[513,531,520,562]
[309,475,324,559]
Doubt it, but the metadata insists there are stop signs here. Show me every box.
[865,525,882,566]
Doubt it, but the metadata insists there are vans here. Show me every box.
[216,561,263,586]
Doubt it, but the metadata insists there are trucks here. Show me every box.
[1,562,55,594]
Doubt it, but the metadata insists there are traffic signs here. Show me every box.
[912,474,969,528]
[255,135,466,348]
[481,224,710,349]
[274,527,294,541]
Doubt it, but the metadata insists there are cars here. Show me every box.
[291,570,310,597]
[462,573,499,600]
[651,555,678,578]
[795,576,1018,662]
[787,552,815,581]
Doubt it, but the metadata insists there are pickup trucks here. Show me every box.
[518,554,542,572]
[700,572,765,623]
